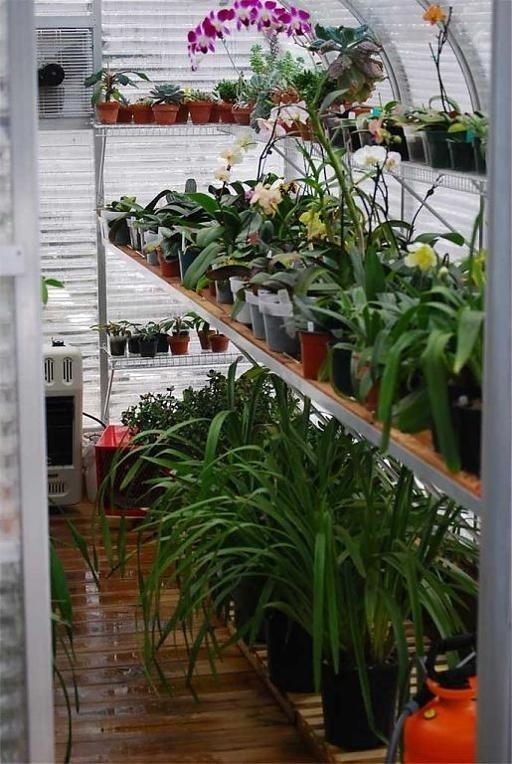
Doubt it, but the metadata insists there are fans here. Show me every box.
[34,27,97,120]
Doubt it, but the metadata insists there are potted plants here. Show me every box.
[239,8,485,175]
[93,387,173,510]
[104,176,232,310]
[170,358,477,746]
[149,82,184,123]
[129,98,152,124]
[84,63,148,122]
[91,320,129,357]
[185,309,213,349]
[164,314,193,355]
[215,79,239,121]
[187,89,215,124]
[129,319,174,355]
[178,89,191,120]
[233,175,485,482]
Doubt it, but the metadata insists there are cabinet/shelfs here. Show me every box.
[97,0,483,763]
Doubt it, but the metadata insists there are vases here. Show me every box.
[211,334,228,353]
